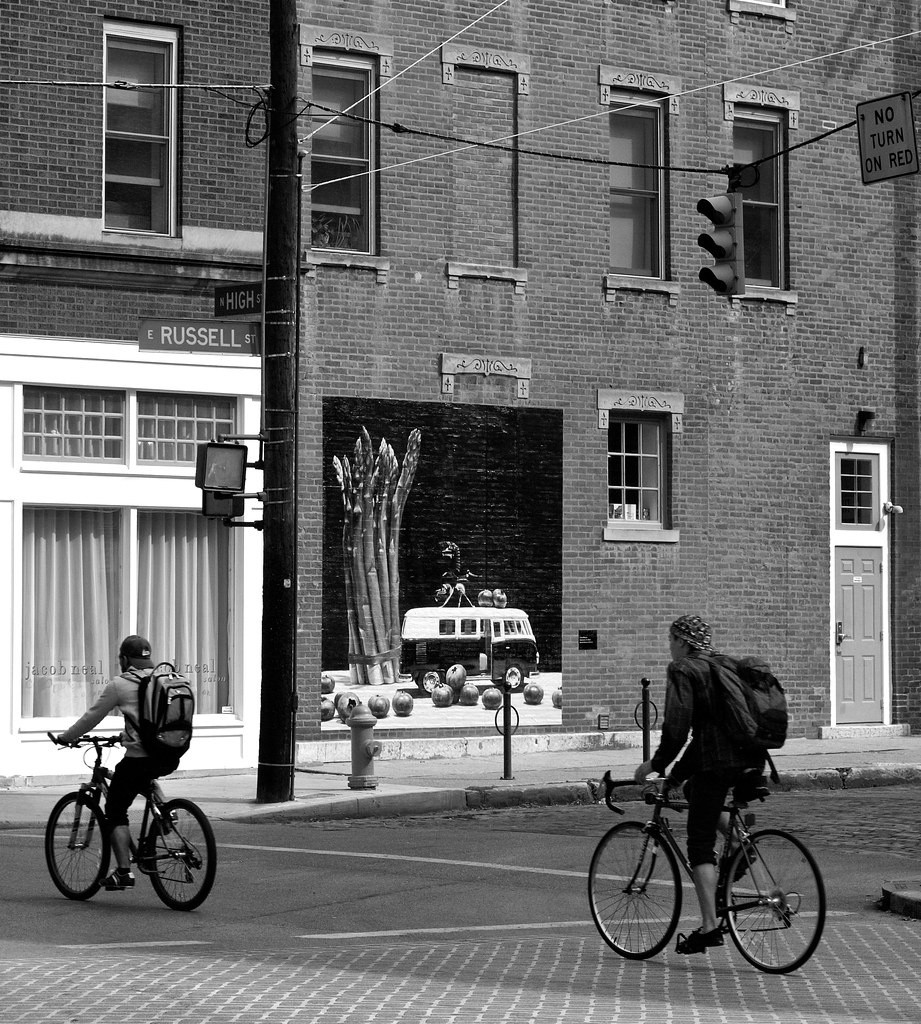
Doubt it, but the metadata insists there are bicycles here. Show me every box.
[44,732,217,911]
[588,770,825,974]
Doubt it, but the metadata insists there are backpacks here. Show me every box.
[682,653,788,749]
[124,662,195,759]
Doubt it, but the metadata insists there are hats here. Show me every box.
[120,635,154,669]
[670,614,718,651]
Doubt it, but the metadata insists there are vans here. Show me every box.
[399,607,540,698]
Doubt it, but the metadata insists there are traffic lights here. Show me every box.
[694,193,747,296]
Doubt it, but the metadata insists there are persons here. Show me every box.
[634,615,766,957]
[57,635,180,889]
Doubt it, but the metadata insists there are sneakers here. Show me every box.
[99,870,135,889]
[678,926,724,954]
[170,809,178,825]
[723,840,757,882]
